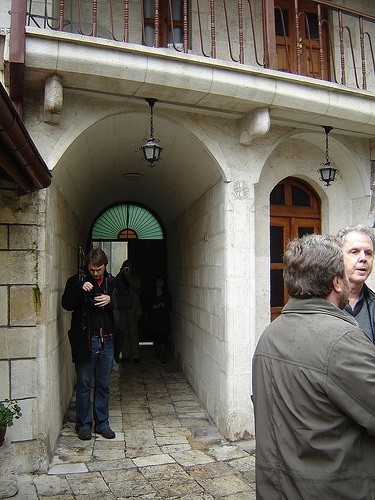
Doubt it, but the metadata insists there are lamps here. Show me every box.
[139,97,164,168]
[317,125,340,187]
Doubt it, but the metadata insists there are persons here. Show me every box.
[336,225,375,344]
[252,235,375,500]
[146,275,172,362]
[61,248,133,440]
[116,260,142,363]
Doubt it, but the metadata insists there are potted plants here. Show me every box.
[0,399,22,447]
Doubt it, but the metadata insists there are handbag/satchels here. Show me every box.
[116,291,133,309]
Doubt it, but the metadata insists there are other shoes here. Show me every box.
[133,355,140,363]
[123,354,131,361]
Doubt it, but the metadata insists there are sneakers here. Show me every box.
[75,424,91,440]
[94,421,115,439]
